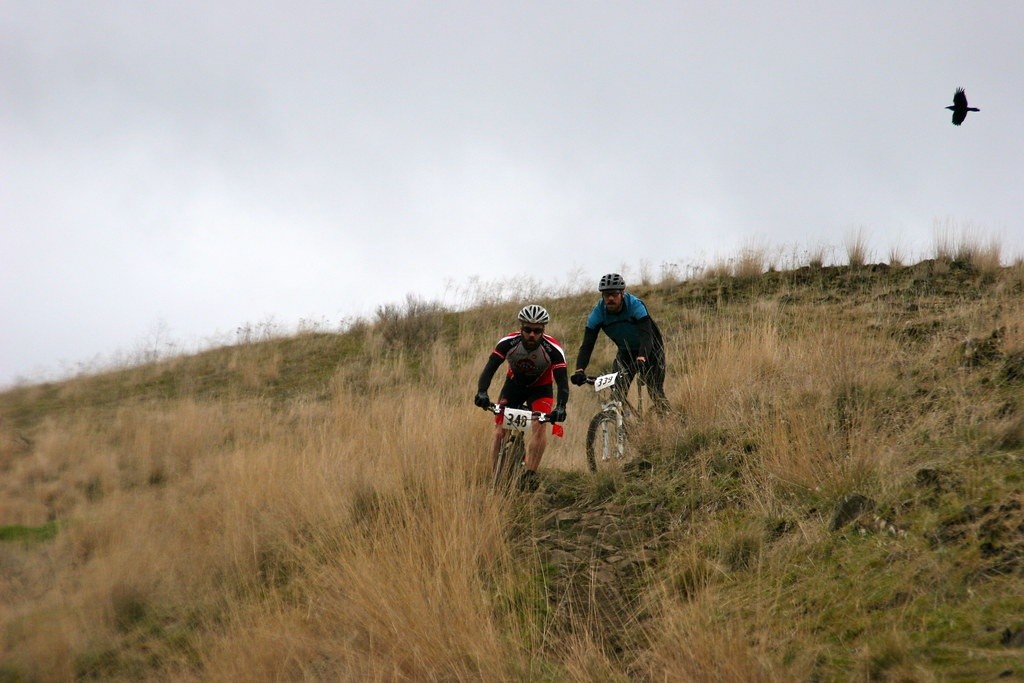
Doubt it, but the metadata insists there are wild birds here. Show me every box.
[945,86,980,127]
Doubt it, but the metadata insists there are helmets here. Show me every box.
[518,304,549,325]
[598,273,625,292]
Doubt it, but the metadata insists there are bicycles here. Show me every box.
[573,364,670,480]
[477,398,566,501]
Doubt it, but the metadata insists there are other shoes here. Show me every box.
[521,469,538,491]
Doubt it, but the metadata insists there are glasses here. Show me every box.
[602,290,622,298]
[521,323,544,334]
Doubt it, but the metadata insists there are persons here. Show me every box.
[474,305,570,494]
[570,273,679,422]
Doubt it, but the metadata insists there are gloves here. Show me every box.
[474,389,490,411]
[549,404,567,425]
[631,360,645,373]
[571,369,586,386]
